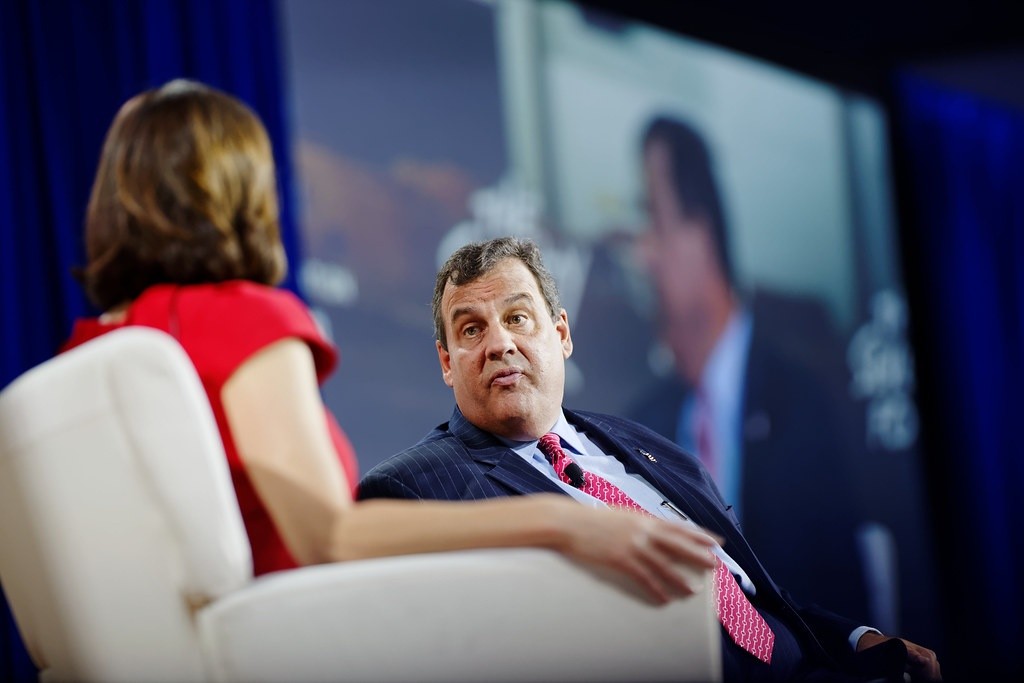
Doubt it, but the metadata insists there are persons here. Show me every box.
[351,235,942,683]
[602,118,917,647]
[62,77,717,606]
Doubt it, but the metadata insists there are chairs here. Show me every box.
[0,325,728,682]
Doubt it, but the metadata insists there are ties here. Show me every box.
[694,400,714,476]
[536,432,775,667]
